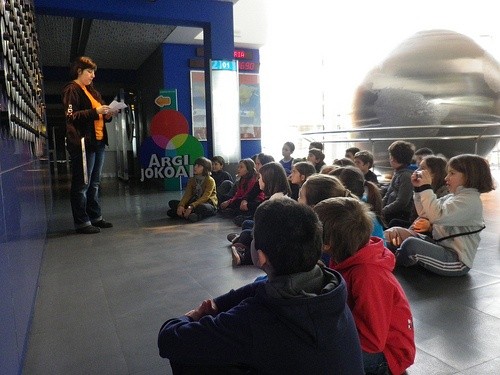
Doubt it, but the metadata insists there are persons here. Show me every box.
[167,157,218,222]
[158,198,365,375]
[62,57,120,234]
[314,197,416,375]
[209,139,450,265]
[328,165,387,247]
[386,154,496,275]
[255,173,359,283]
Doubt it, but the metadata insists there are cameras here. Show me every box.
[415,171,422,180]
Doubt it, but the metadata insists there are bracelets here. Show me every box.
[187,205,193,209]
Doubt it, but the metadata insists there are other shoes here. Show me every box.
[231,246,246,265]
[190,213,201,222]
[75,225,100,234]
[92,220,112,228]
[227,233,238,242]
[167,210,176,217]
[234,243,246,248]
